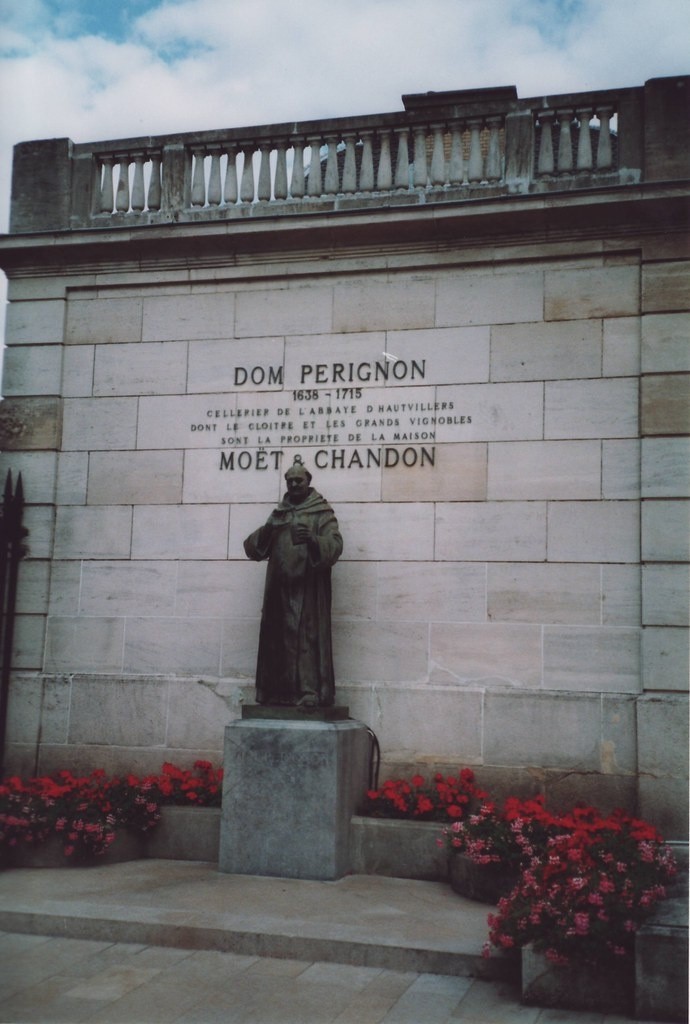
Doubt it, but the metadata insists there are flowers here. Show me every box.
[367,768,677,966]
[0,760,223,860]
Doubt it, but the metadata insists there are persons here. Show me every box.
[244,467,343,704]
